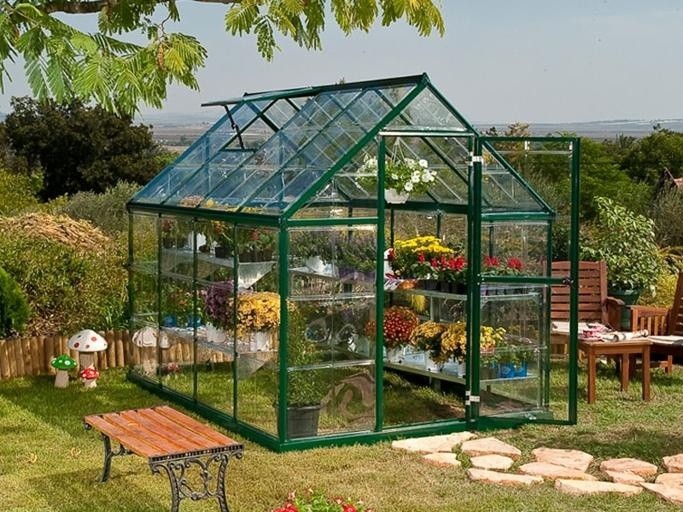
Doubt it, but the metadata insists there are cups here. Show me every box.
[581,323,604,339]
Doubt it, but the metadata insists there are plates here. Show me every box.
[577,335,604,343]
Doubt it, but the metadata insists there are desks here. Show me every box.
[80,403,245,512]
[575,329,656,404]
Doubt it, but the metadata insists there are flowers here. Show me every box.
[387,235,451,268]
[354,153,439,191]
[419,251,466,271]
[479,325,507,355]
[268,487,376,511]
[365,305,417,348]
[229,289,296,345]
[409,318,444,349]
[485,256,524,271]
[203,280,236,326]
[442,321,467,353]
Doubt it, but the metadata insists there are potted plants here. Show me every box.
[272,306,327,437]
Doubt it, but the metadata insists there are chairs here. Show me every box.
[541,257,683,374]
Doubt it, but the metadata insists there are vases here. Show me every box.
[248,333,273,351]
[203,323,227,344]
[423,349,445,370]
[457,357,466,378]
[384,188,409,204]
[384,344,406,363]
